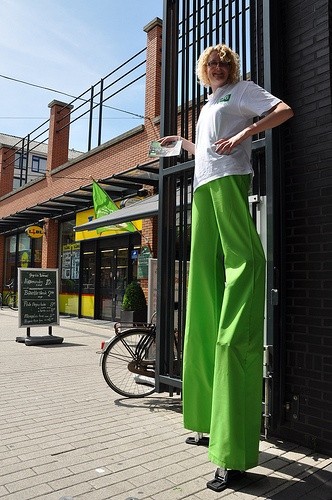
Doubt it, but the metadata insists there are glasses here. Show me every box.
[205,60,229,67]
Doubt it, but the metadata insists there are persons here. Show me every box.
[156,44,292,347]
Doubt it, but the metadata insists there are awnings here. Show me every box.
[73,185,191,232]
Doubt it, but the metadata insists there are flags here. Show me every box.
[93,179,134,234]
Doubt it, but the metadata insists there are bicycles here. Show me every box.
[96,302,177,398]
[0,278,18,311]
